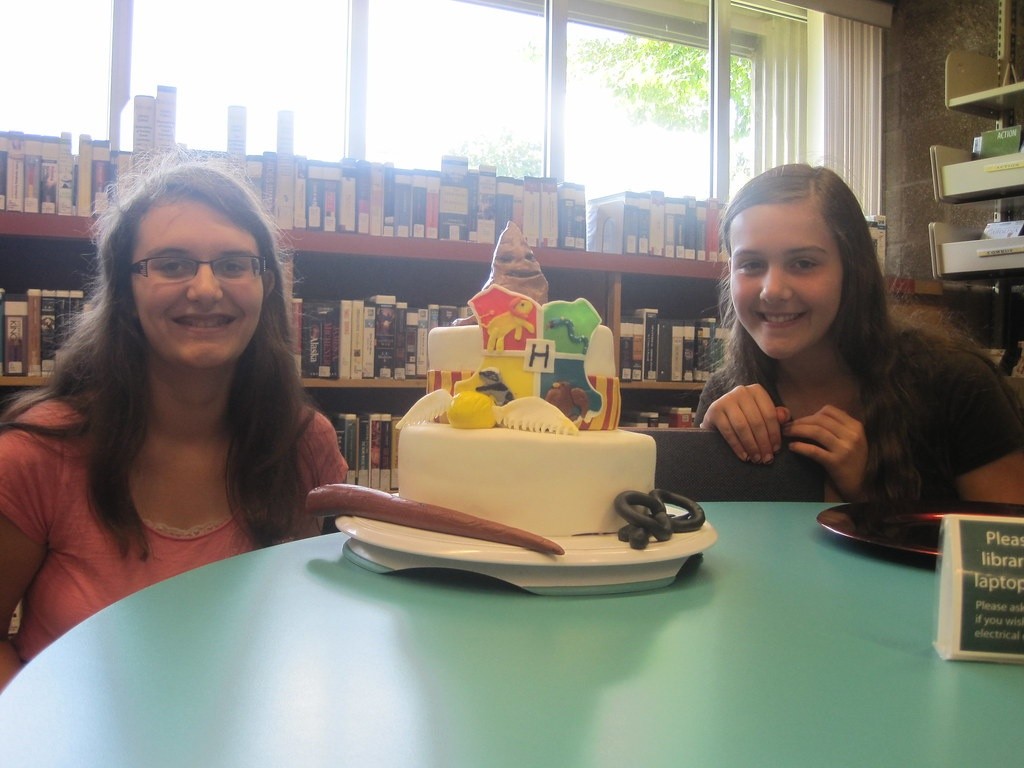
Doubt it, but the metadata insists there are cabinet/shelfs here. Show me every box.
[927,51,1024,349]
[0,211,728,645]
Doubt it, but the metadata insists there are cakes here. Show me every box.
[394,218,657,539]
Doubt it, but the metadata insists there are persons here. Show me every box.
[0,159,353,697]
[694,162,1023,506]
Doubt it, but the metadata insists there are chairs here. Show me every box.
[618,426,825,502]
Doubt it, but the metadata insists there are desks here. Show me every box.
[0,502,1024,768]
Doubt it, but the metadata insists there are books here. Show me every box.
[620,309,733,382]
[325,414,406,492]
[0,84,734,262]
[625,406,700,429]
[865,212,886,278]
[2,288,98,377]
[290,292,473,380]
[618,423,825,504]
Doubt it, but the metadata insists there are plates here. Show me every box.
[815,500,1024,568]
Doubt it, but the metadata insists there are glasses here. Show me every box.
[131,255,268,281]
